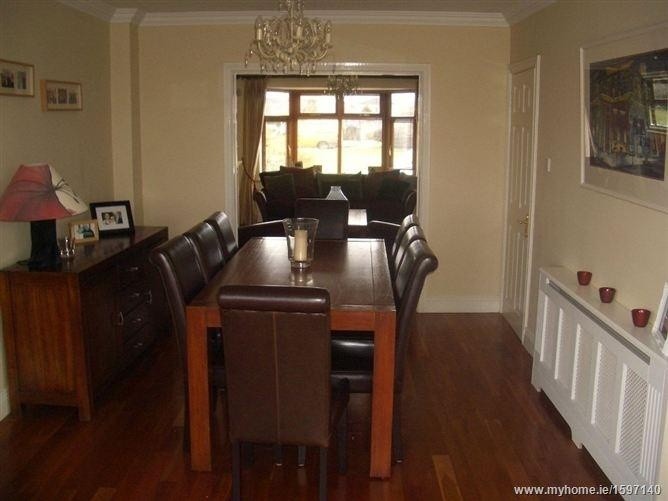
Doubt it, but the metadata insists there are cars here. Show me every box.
[295,127,339,150]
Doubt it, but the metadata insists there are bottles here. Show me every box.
[326,186,349,201]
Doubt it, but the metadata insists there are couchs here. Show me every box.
[251,165,417,223]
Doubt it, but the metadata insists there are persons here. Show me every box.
[81,225,94,238]
[103,211,123,225]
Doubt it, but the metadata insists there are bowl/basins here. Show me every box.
[45,248,81,267]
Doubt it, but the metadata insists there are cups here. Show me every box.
[282,217,319,269]
[288,269,313,288]
[599,287,616,303]
[577,271,592,286]
[56,231,76,258]
[631,308,651,328]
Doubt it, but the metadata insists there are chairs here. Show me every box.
[144,200,438,499]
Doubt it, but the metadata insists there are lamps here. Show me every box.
[323,74,362,99]
[0,164,89,272]
[242,1,332,77]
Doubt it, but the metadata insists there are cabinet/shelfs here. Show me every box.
[0,227,168,423]
[531,262,665,500]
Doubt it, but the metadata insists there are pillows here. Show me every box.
[261,164,417,209]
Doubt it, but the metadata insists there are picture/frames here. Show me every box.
[39,79,82,113]
[579,22,668,212]
[651,281,668,360]
[88,200,136,238]
[0,59,36,98]
[68,220,99,244]
